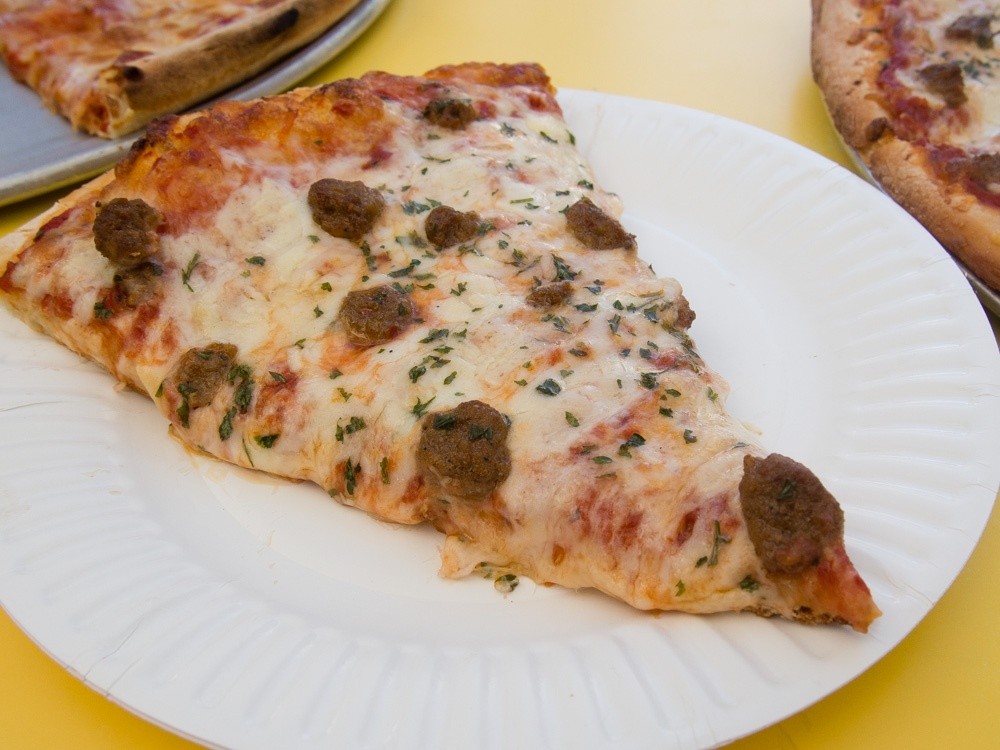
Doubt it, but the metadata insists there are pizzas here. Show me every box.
[0,62,882,638]
[1,0,365,139]
[808,0,1000,300]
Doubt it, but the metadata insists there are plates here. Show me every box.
[0,94,1000,749]
[0,0,390,203]
